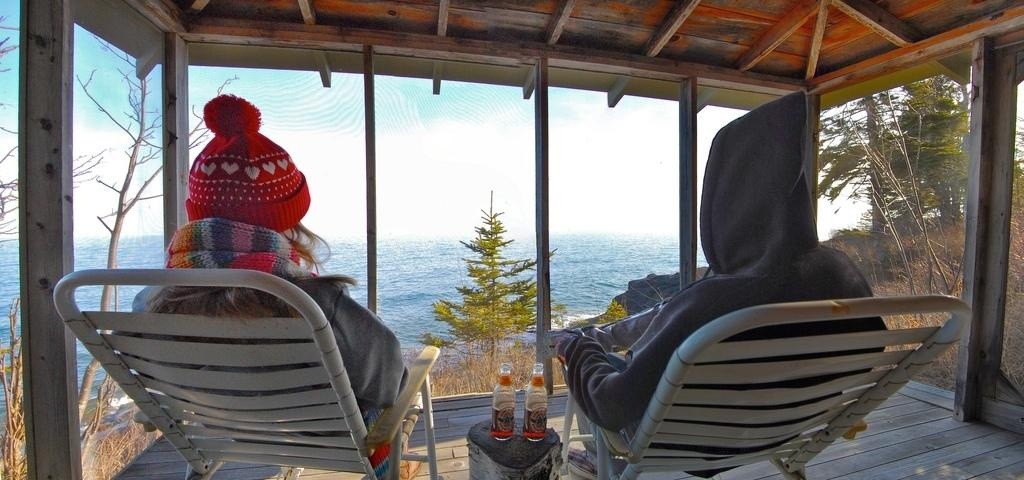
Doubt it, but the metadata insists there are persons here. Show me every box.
[125,93,407,480]
[555,90,889,480]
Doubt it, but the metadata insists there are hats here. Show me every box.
[184,92,311,235]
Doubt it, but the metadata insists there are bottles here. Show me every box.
[523,362,549,441]
[491,364,515,441]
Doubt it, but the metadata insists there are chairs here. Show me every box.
[566,287,969,480]
[49,264,445,480]
